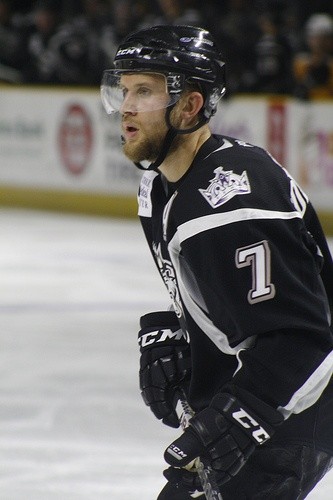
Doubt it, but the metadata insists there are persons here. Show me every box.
[0,0,333,100]
[101,24,333,500]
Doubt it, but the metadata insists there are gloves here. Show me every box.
[137,310,190,430]
[162,385,287,492]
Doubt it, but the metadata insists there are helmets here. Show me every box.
[113,26,225,118]
